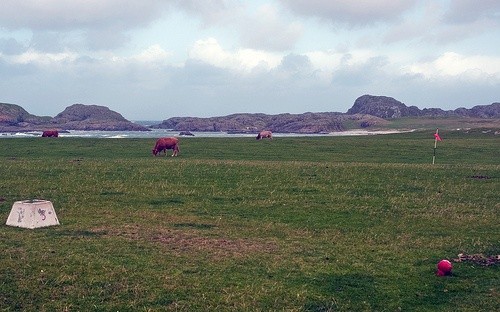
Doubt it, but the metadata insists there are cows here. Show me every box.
[256,130,273,144]
[152,137,180,158]
[41,130,59,138]
